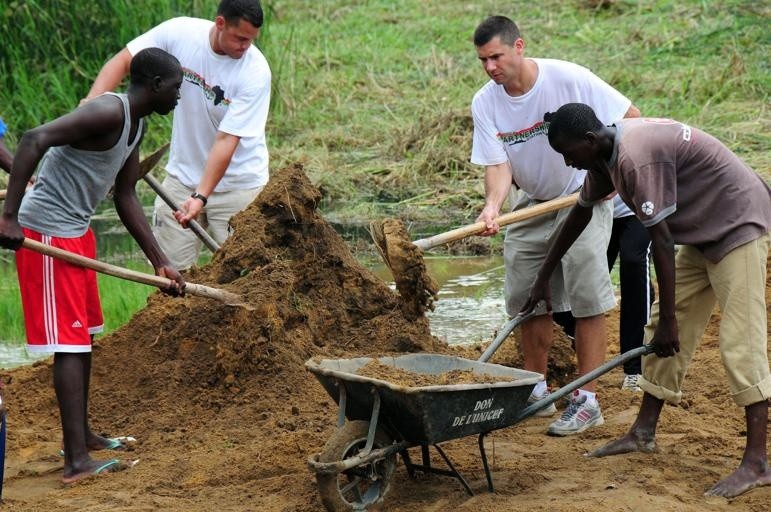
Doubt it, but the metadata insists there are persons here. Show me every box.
[0,48,186,484]
[0,115,36,187]
[605,191,656,393]
[469,15,643,436]
[518,102,771,499]
[72,1,270,275]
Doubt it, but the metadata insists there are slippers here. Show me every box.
[65,458,140,489]
[59,435,136,456]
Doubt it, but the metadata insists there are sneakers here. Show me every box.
[547,400,606,435]
[524,388,558,419]
[620,374,643,392]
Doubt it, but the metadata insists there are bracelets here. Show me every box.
[188,188,209,208]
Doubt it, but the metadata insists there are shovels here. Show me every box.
[22,237,294,311]
[369,191,580,271]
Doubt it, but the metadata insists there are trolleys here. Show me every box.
[304,307,657,512]
